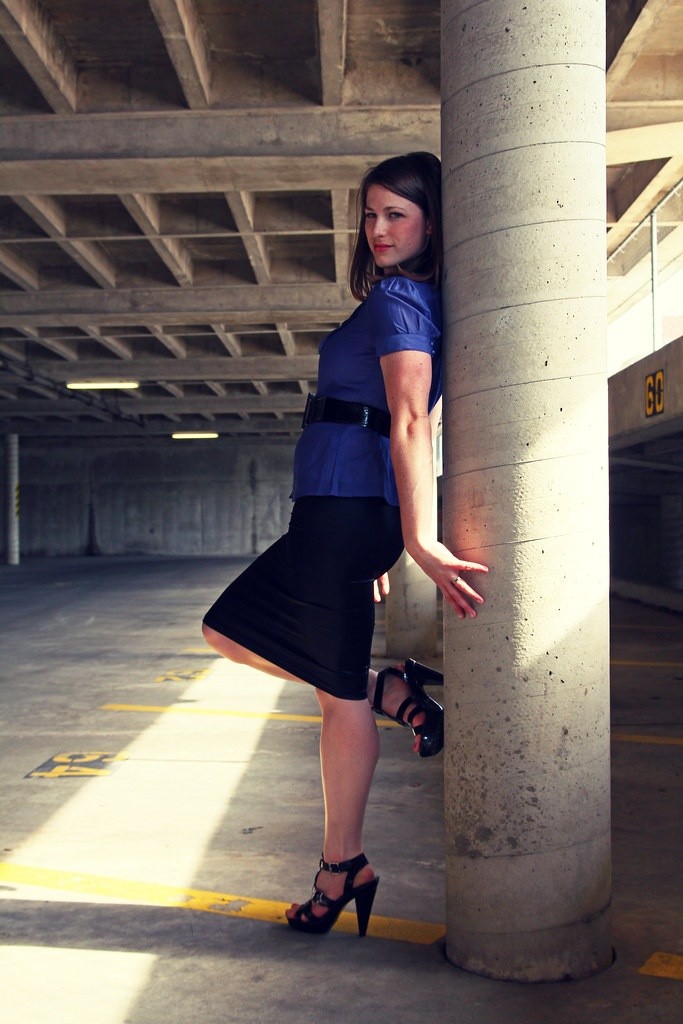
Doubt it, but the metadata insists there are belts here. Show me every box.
[301,393,392,440]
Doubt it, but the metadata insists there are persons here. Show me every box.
[201,152,490,936]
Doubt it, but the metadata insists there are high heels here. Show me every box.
[371,658,444,757]
[287,851,380,937]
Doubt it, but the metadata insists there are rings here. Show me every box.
[453,577,461,584]
